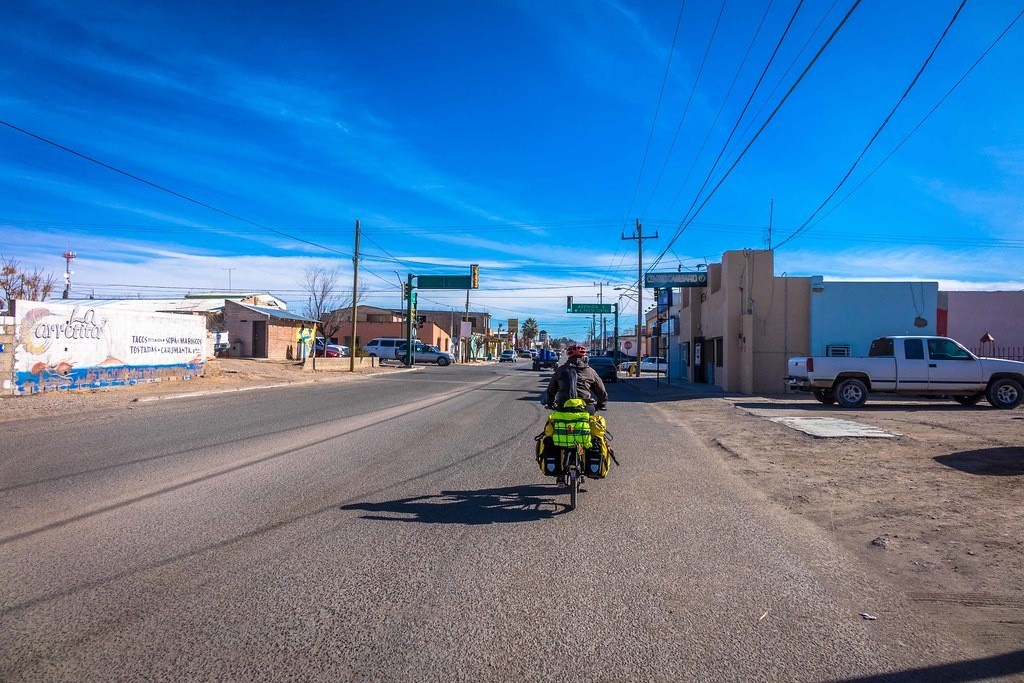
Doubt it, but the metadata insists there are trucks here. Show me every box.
[532,349,558,371]
[589,350,637,365]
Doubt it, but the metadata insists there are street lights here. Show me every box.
[585,317,603,350]
[614,288,642,377]
[394,270,403,338]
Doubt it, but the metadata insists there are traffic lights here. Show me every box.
[418,317,423,328]
[567,296,573,313]
[404,282,408,300]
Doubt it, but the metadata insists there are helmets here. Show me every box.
[565,344,586,356]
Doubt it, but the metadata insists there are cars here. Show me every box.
[621,357,668,374]
[316,337,350,357]
[521,349,537,358]
[395,343,455,366]
[499,349,518,362]
[316,345,339,358]
[588,357,617,383]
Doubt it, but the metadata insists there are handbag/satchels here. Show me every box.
[535,416,567,476]
[581,415,611,478]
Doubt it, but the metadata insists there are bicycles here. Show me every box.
[545,404,607,510]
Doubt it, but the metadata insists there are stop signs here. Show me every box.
[624,341,632,350]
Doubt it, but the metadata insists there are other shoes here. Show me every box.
[580,475,586,484]
[556,476,567,488]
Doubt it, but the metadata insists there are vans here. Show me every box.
[363,338,421,362]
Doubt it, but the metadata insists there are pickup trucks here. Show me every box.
[783,335,1024,409]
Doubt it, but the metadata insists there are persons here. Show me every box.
[546,344,608,411]
